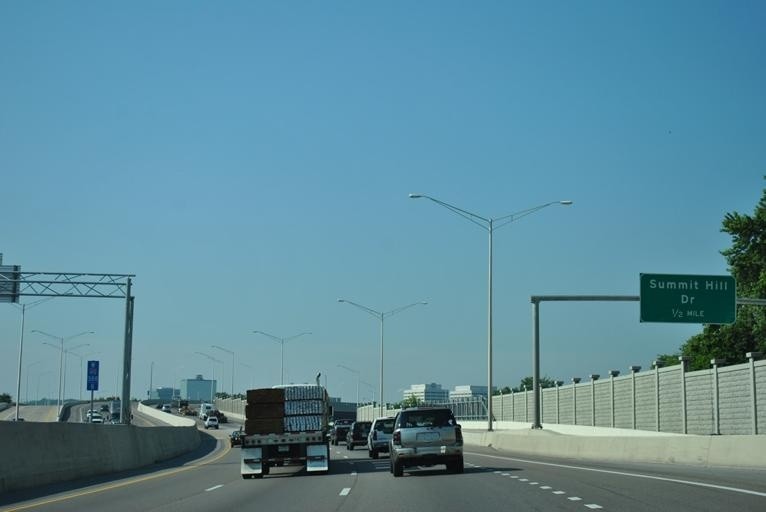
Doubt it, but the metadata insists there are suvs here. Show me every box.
[346,422,372,451]
[388,408,462,478]
[367,417,395,460]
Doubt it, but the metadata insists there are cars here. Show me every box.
[331,420,353,445]
[86,398,134,425]
[326,422,335,440]
[229,430,245,447]
[157,396,227,430]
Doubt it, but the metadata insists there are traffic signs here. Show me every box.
[641,274,736,324]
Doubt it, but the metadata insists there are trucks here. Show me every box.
[240,385,333,479]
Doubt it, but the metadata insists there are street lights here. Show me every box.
[253,331,312,386]
[335,364,375,404]
[42,343,89,405]
[408,192,573,433]
[194,351,223,395]
[211,344,234,396]
[66,351,99,400]
[23,360,39,403]
[149,361,154,400]
[171,375,189,396]
[4,292,72,421]
[336,298,428,420]
[31,330,95,417]
[34,371,55,405]
